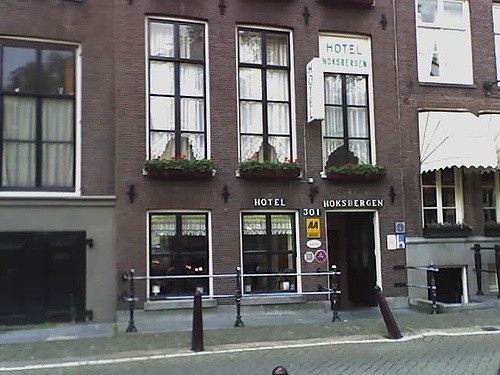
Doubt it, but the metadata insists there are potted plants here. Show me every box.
[142,154,218,178]
[423,222,473,240]
[326,162,387,182]
[484,221,500,237]
[234,158,303,177]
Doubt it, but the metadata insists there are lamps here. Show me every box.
[483,80,500,90]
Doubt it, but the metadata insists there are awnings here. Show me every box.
[418,112,500,174]
